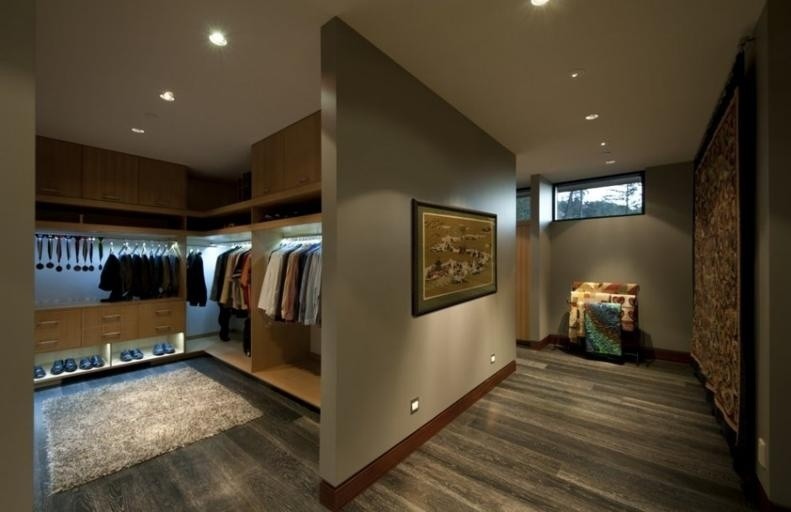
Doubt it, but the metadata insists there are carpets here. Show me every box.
[38,365,264,497]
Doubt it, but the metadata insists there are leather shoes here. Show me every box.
[33,366,45,378]
[120,348,143,361]
[50,355,104,375]
[153,342,175,355]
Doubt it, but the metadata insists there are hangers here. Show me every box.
[268,233,322,259]
[216,240,252,262]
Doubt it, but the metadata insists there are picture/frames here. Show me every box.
[410,197,499,318]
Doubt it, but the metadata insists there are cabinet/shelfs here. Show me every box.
[32,297,186,389]
[34,108,322,216]
